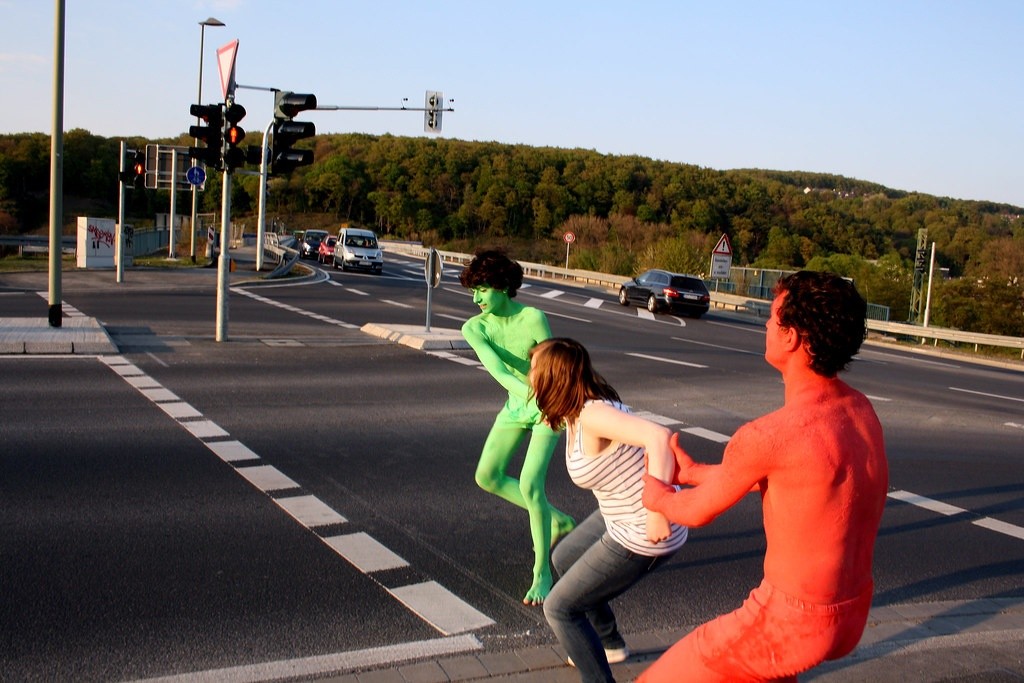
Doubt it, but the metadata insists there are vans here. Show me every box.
[333,228,385,273]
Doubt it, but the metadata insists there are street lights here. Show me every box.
[271,216,279,232]
[191,17,226,264]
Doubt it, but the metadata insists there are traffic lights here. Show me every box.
[915,250,927,273]
[271,91,317,177]
[189,104,222,167]
[225,105,246,167]
[132,153,145,188]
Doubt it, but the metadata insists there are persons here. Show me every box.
[366,240,371,248]
[634,270,892,683]
[528,337,690,682]
[347,237,354,246]
[459,250,575,607]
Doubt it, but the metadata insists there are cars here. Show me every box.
[618,269,710,319]
[291,231,304,240]
[298,230,329,259]
[318,235,338,264]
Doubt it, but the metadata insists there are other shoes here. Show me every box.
[567,645,628,666]
